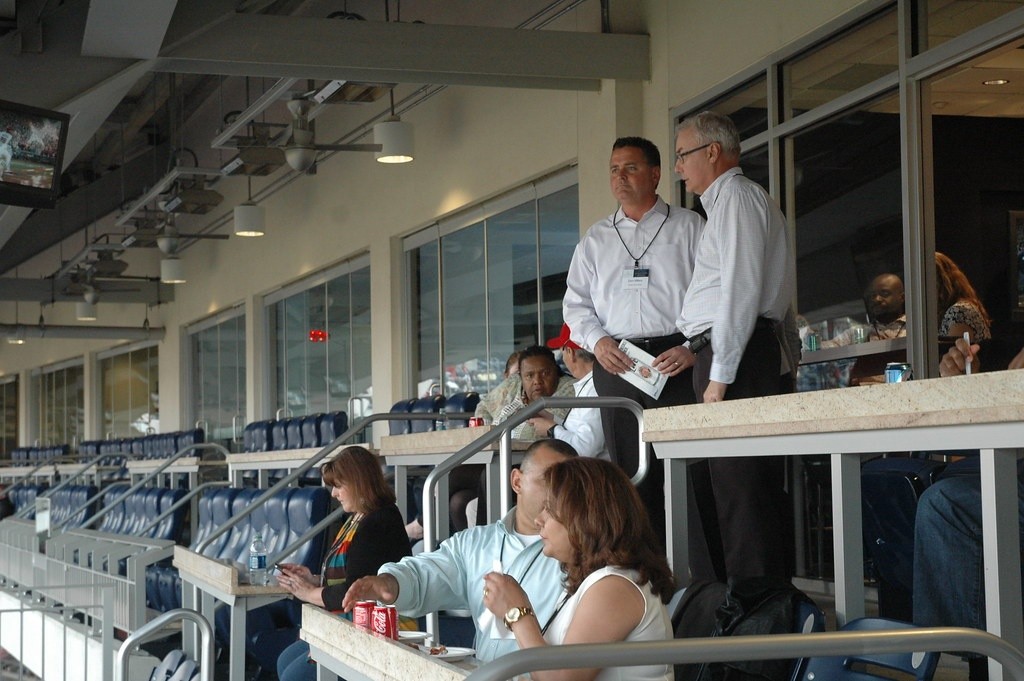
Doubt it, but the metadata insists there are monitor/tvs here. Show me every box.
[0,98,70,210]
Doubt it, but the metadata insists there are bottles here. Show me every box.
[247,530,267,586]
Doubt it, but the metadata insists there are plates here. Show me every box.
[398,630,433,643]
[421,646,475,662]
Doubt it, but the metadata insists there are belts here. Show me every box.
[689,318,773,354]
[630,333,682,351]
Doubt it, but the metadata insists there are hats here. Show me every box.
[547,322,583,350]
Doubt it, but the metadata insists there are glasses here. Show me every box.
[676,144,712,164]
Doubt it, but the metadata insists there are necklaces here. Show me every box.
[350,520,354,524]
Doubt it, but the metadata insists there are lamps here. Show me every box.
[230,175,267,239]
[74,301,99,321]
[159,254,187,284]
[154,201,180,254]
[374,84,418,165]
[283,97,317,175]
[80,263,103,306]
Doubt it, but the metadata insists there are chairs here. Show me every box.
[0,384,943,681]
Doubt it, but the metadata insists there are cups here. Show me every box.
[853,325,865,344]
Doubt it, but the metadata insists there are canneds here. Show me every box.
[808,331,821,351]
[371,604,399,642]
[468,416,484,427]
[883,362,913,383]
[352,600,377,629]
[854,325,868,344]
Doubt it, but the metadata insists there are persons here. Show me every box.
[561,111,793,584]
[342,438,678,681]
[276,446,413,681]
[404,322,611,542]
[796,252,992,388]
[911,338,1024,658]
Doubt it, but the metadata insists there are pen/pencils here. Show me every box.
[963,331,972,375]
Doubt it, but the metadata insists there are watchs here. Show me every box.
[503,607,535,632]
[547,424,559,438]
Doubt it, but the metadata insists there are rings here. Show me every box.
[484,588,489,598]
[675,363,679,366]
[287,581,291,585]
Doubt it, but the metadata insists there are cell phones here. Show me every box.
[273,562,287,574]
